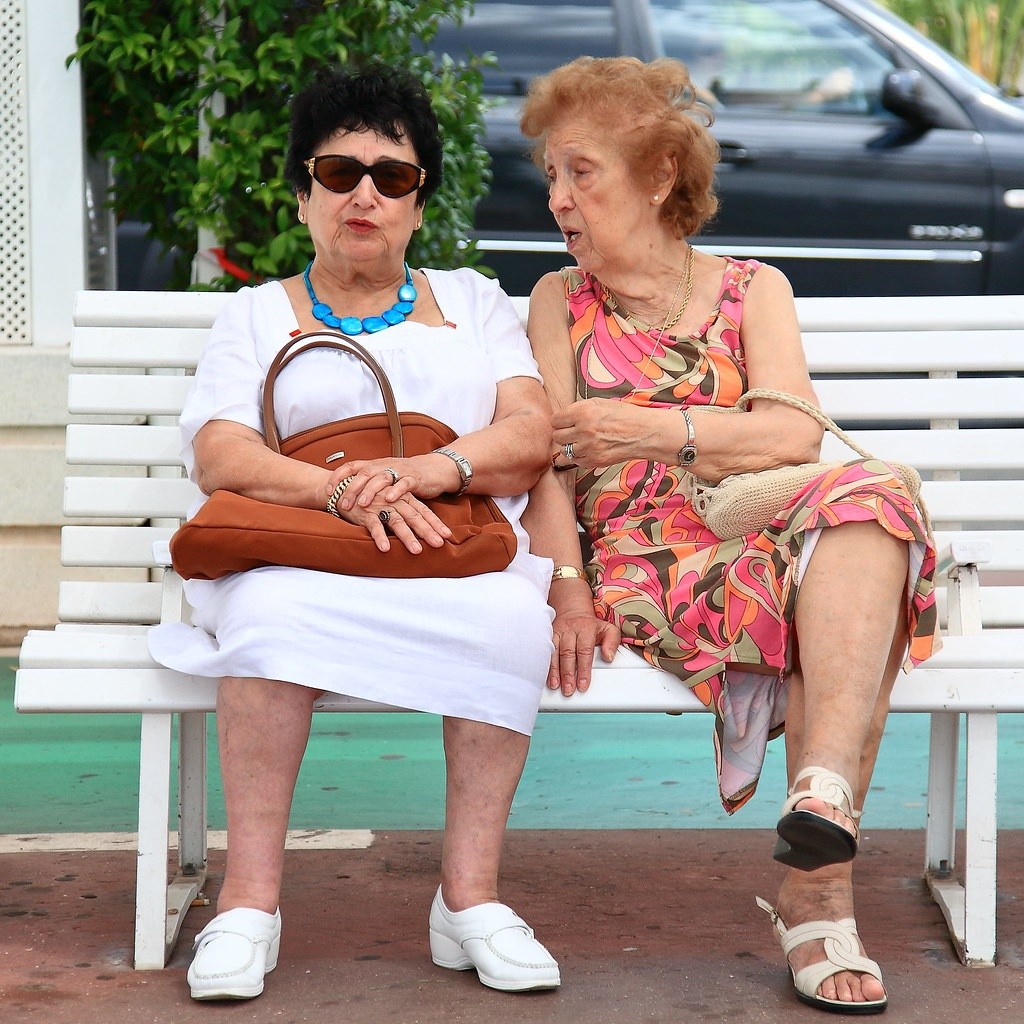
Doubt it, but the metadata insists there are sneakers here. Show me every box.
[187,905,282,1000]
[429,883,561,992]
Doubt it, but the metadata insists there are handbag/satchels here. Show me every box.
[687,389,936,550]
[168,332,517,581]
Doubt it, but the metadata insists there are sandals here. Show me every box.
[773,767,866,872]
[755,896,889,1015]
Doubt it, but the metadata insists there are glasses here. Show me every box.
[304,155,428,198]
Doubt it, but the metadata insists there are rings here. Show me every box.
[386,468,398,482]
[379,510,397,523]
[566,443,576,459]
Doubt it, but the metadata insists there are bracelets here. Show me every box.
[327,476,357,517]
[552,565,592,592]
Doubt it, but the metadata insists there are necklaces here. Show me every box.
[304,262,417,336]
[602,247,695,330]
[585,245,692,402]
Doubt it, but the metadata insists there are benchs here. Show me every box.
[15,289,1023,969]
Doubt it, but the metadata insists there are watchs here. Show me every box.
[431,448,473,496]
[679,411,697,466]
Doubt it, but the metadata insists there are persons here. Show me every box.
[145,62,562,1001]
[520,57,942,1015]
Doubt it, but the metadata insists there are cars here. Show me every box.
[397,0,1024,298]
